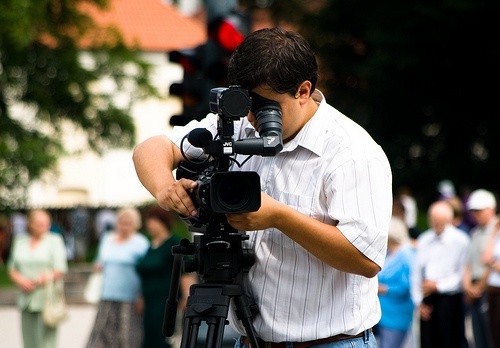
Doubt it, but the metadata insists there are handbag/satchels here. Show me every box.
[83,271,103,306]
[41,273,67,329]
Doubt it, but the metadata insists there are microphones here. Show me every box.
[186,128,213,148]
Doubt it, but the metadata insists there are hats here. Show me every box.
[466,189,495,211]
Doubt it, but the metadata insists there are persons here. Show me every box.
[7,210,69,348]
[135,206,197,348]
[375,189,500,348]
[134,28,392,348]
[85,207,148,348]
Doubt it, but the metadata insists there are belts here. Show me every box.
[239,328,373,348]
